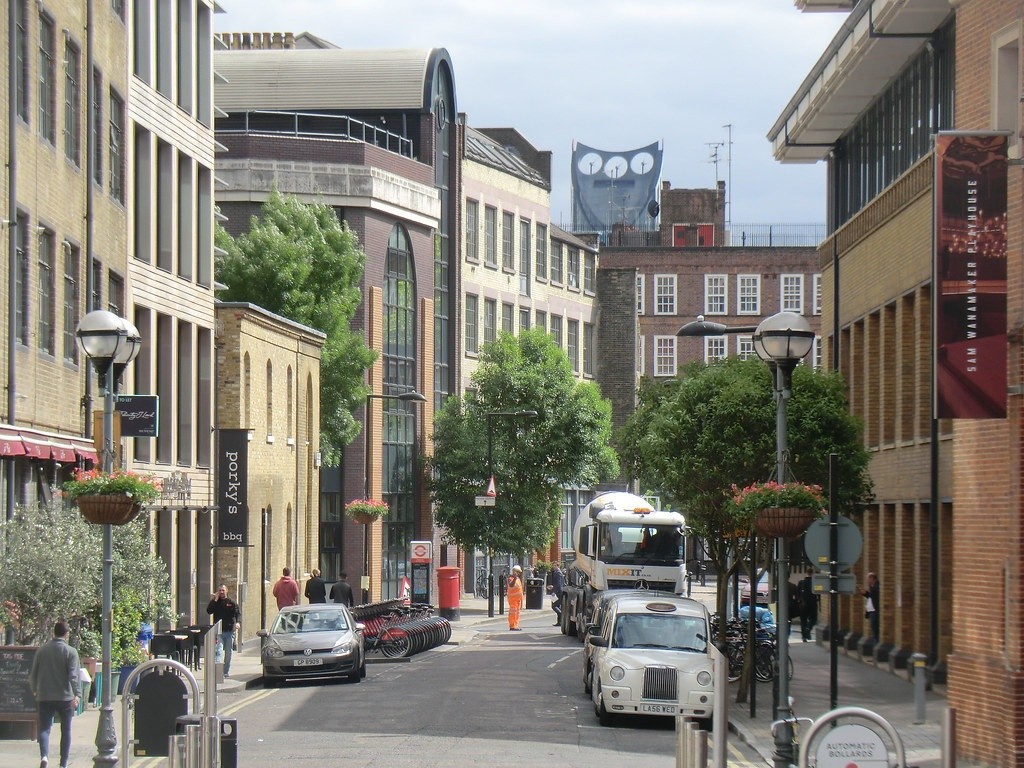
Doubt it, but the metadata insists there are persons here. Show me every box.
[206,583,242,679]
[601,527,626,556]
[304,568,327,604]
[506,564,523,631]
[787,569,820,643]
[273,567,300,611]
[547,561,565,626]
[329,572,354,608]
[30,620,82,768]
[856,572,879,644]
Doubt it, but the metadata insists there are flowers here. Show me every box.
[63,467,163,509]
[722,481,828,523]
[343,499,391,527]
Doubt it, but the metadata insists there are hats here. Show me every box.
[513,564,522,573]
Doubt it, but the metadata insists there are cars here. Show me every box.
[740,568,769,609]
[256,603,366,688]
[582,589,714,727]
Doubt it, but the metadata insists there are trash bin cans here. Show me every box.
[527,578,545,610]
[174,714,238,768]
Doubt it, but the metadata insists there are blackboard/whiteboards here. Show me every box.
[0,646,40,722]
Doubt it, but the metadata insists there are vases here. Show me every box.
[755,508,815,538]
[77,495,142,525]
[353,510,378,524]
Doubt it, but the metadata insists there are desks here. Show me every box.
[165,630,201,670]
[173,634,188,676]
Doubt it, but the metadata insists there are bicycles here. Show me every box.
[348,596,452,658]
[476,565,489,599]
[710,611,793,683]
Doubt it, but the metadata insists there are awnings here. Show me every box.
[0,427,100,464]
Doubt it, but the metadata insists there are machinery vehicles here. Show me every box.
[559,490,701,647]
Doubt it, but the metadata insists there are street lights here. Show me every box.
[72,309,143,768]
[676,310,780,719]
[487,409,538,617]
[361,390,427,605]
[752,313,815,768]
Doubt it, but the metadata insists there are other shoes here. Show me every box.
[803,639,807,643]
[806,628,811,640]
[224,673,230,678]
[554,623,561,626]
[510,627,522,631]
[40,757,47,768]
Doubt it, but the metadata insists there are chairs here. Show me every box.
[170,630,198,672]
[150,634,180,676]
